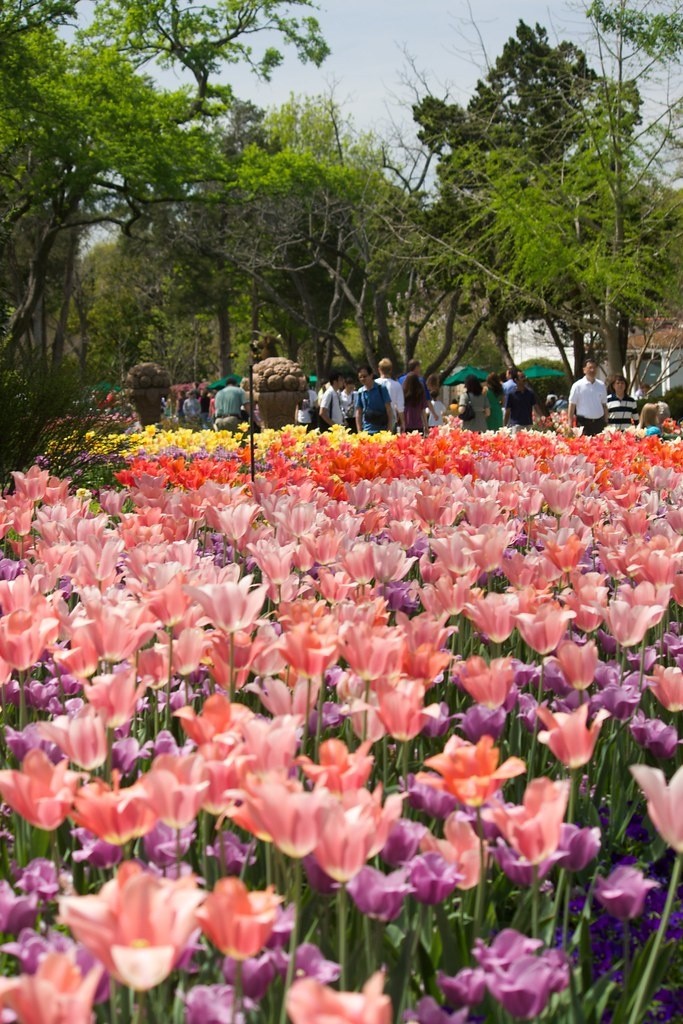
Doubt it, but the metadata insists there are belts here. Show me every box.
[217,413,237,418]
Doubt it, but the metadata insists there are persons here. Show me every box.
[545,393,567,413]
[426,373,440,421]
[295,372,359,434]
[176,378,259,438]
[354,365,394,436]
[374,358,405,435]
[635,400,662,439]
[568,358,610,437]
[607,376,640,432]
[402,372,430,434]
[425,388,446,429]
[397,360,439,422]
[449,399,459,414]
[633,384,651,401]
[502,368,546,431]
[482,370,505,431]
[457,374,491,434]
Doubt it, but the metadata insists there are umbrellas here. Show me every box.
[206,373,243,391]
[444,365,489,385]
[523,365,567,379]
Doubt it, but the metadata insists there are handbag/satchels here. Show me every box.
[345,392,354,418]
[364,409,388,425]
[458,391,476,422]
[318,415,331,433]
